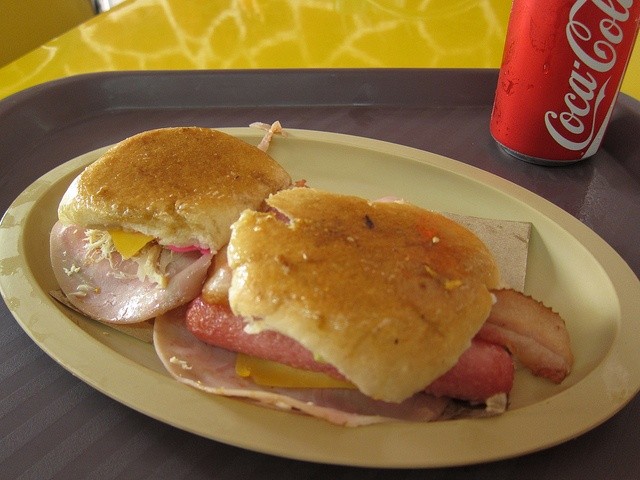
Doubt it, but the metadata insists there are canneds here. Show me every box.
[489,0,640,170]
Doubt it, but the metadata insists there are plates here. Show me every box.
[1,126,640,471]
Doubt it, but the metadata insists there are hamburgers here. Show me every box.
[50,125,294,327]
[150,188,571,421]
